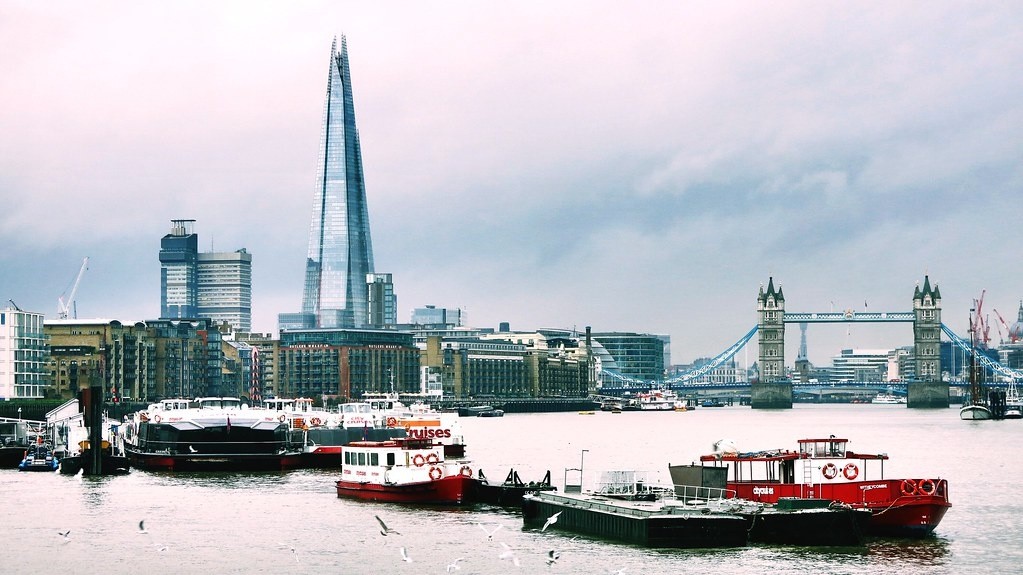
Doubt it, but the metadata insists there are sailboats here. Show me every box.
[959,313,993,421]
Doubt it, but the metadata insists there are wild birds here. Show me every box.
[74,468,84,484]
[542,511,562,532]
[478,523,503,542]
[499,541,521,567]
[189,445,198,453]
[545,550,560,566]
[447,558,463,572]
[375,515,403,536]
[58,531,71,537]
[401,547,412,562]
[136,520,148,534]
[281,542,299,563]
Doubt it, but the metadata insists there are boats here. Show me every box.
[871,392,907,405]
[334,419,489,508]
[993,372,1023,420]
[588,379,726,412]
[700,435,953,541]
[457,405,494,417]
[476,468,559,509]
[477,409,505,418]
[17,397,469,477]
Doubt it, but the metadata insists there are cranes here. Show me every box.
[57,257,90,319]
[970,289,1023,350]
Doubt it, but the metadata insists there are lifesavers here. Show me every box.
[428,467,442,480]
[388,417,396,426]
[843,464,858,480]
[900,479,917,495]
[823,463,838,479]
[280,415,285,422]
[154,415,161,423]
[413,454,424,467]
[310,418,321,425]
[918,479,935,495]
[426,453,438,465]
[460,466,473,477]
